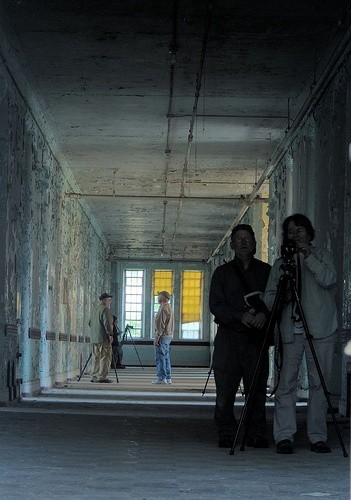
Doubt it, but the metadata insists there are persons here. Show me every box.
[208,224,277,450]
[88,293,114,383]
[111,315,126,369]
[263,213,340,455]
[152,291,176,384]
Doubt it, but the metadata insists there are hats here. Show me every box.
[99,293,113,301]
[157,291,171,300]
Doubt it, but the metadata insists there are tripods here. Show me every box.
[228,264,348,458]
[78,348,119,383]
[117,326,145,369]
[202,324,244,397]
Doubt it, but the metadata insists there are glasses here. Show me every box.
[235,236,253,243]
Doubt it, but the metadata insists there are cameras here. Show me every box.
[280,239,304,264]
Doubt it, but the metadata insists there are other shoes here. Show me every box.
[90,378,113,383]
[214,424,243,448]
[152,377,172,384]
[276,438,297,454]
[310,439,332,454]
[244,422,272,448]
[111,364,126,369]
